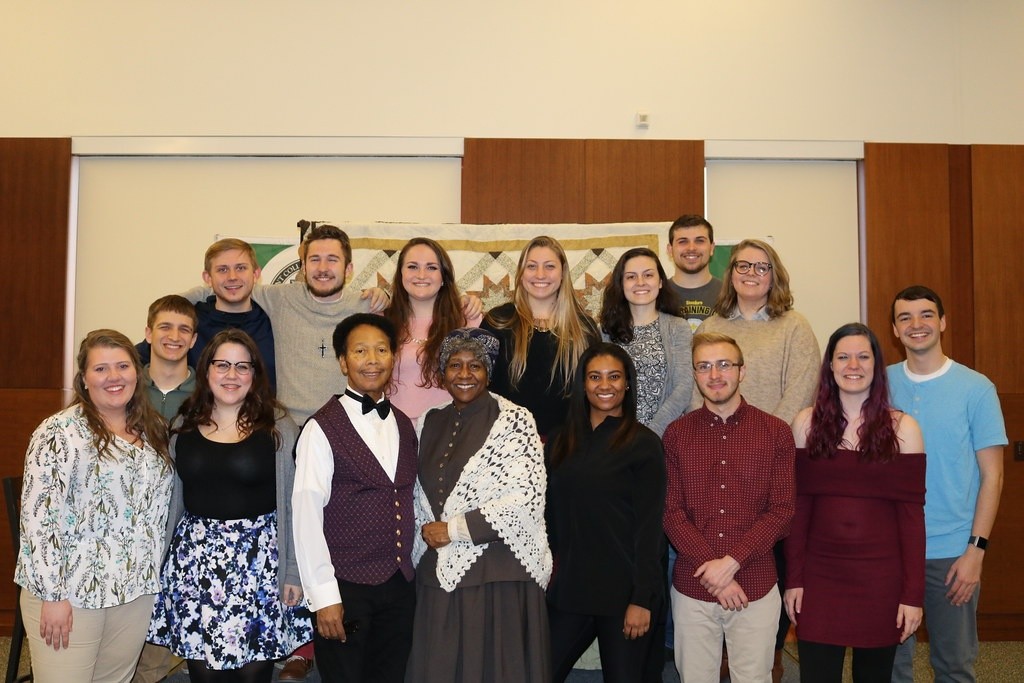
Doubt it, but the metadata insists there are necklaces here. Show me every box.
[318,339,327,358]
[216,422,235,433]
[411,338,427,344]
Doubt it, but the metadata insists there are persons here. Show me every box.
[479,236,603,601]
[403,327,553,683]
[134,238,390,673]
[663,332,796,683]
[684,239,822,683]
[885,285,1008,683]
[138,295,199,422]
[13,329,174,683]
[784,323,926,683]
[291,312,418,683]
[144,329,314,683]
[544,342,669,683]
[378,238,485,430]
[597,248,694,660]
[178,225,483,683]
[667,214,723,334]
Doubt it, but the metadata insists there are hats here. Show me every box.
[439,327,500,380]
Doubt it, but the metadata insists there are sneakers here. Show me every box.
[720,648,729,680]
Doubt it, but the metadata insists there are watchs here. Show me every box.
[968,536,988,550]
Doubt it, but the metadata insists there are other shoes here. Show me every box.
[773,648,783,683]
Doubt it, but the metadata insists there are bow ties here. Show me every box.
[345,387,390,420]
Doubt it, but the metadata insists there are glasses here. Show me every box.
[695,361,739,373]
[731,261,772,276]
[210,360,254,375]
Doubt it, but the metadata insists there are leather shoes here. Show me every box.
[278,655,313,683]
